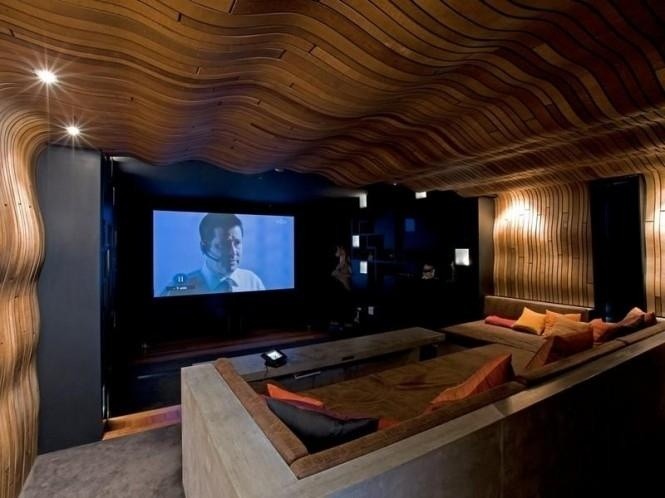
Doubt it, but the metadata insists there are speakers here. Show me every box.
[583,174,649,323]
[34,145,118,455]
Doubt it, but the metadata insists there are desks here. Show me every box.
[192,325,448,388]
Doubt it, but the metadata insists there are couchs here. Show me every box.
[440,296,592,349]
[180,309,665,498]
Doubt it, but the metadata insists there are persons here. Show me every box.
[160,213,267,297]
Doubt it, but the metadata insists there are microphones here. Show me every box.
[201,244,219,263]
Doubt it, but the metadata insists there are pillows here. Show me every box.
[484,306,655,370]
[263,382,402,455]
[418,350,517,416]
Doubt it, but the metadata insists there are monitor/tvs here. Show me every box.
[150,207,297,300]
